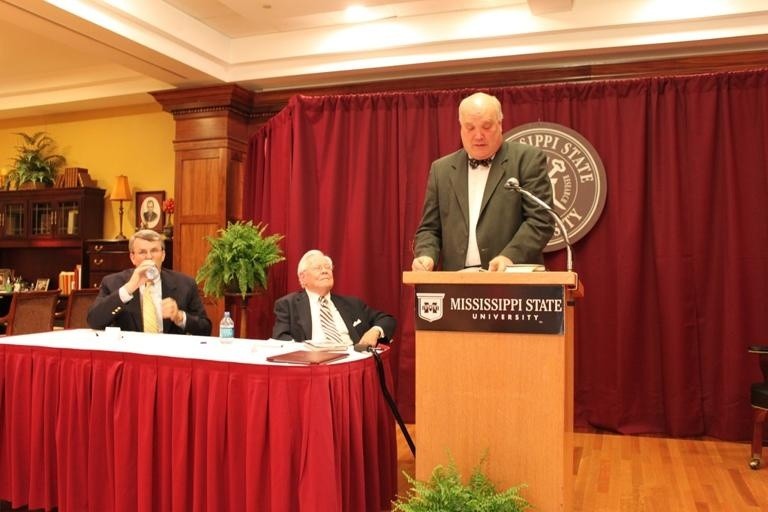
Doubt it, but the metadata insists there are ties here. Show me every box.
[318,296,346,347]
[142,280,159,332]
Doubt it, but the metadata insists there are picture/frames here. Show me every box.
[135,191,167,233]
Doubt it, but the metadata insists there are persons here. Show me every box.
[411,90,556,272]
[271,249,398,348]
[143,199,160,222]
[87,228,212,336]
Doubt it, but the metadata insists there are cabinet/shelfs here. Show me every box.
[82,239,173,287]
[0,188,105,288]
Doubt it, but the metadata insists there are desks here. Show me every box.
[1,327,393,512]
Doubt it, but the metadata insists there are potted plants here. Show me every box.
[6,130,66,189]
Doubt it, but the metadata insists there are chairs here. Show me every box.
[1,288,62,337]
[745,346,768,467]
[63,287,103,328]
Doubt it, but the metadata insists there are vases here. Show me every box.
[162,214,174,240]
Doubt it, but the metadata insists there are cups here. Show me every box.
[146,264,160,282]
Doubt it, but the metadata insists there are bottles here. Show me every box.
[5,276,25,292]
[219,312,234,344]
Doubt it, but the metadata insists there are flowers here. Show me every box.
[162,197,174,214]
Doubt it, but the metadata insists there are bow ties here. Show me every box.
[470,159,491,169]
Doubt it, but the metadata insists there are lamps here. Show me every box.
[109,175,133,240]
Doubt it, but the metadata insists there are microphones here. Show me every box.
[504,177,573,273]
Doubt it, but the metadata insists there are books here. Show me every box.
[269,350,348,364]
[0,165,96,296]
